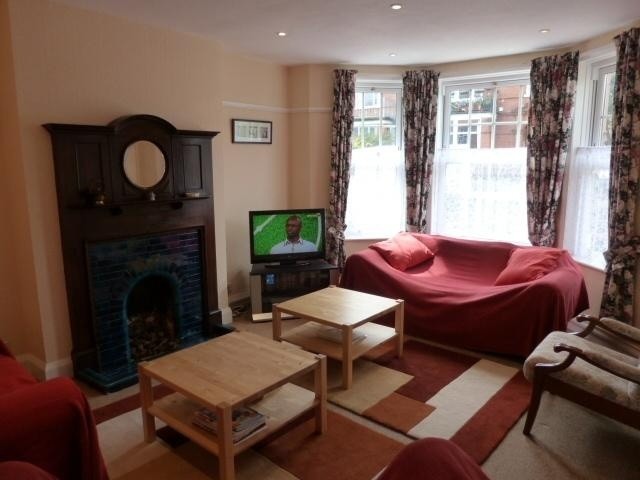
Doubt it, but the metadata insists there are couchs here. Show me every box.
[0,337,101,479]
[341,234,584,366]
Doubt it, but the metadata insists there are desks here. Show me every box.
[248,258,339,322]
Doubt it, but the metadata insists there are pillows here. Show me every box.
[494,248,567,284]
[369,233,436,271]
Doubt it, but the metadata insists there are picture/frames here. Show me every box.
[231,118,273,145]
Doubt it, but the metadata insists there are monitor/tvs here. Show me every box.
[248,208,327,269]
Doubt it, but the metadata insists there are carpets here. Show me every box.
[103,406,406,480]
[288,335,536,469]
[66,373,406,480]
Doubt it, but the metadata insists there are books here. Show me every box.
[192,405,271,447]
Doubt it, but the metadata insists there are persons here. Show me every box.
[270,216,317,253]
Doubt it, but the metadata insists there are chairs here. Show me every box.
[523,314,639,435]
